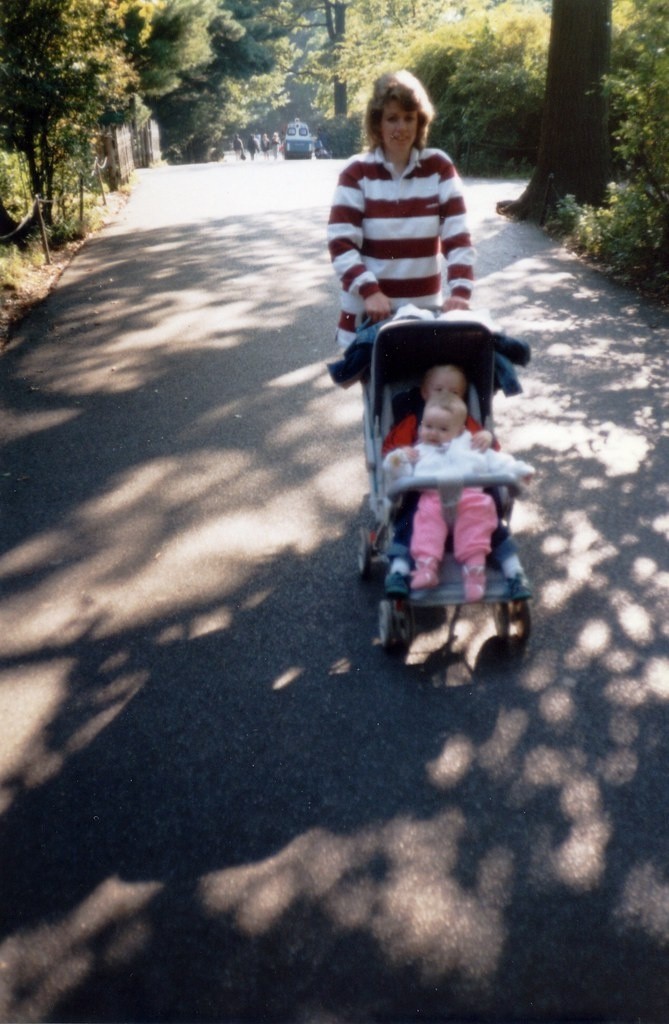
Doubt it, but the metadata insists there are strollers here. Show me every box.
[327,303,531,652]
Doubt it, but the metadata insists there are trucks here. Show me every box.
[284,122,313,158]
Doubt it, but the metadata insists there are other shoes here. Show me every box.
[385,571,410,599]
[409,557,439,589]
[463,565,486,602]
[503,572,531,600]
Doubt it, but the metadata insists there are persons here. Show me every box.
[261,134,270,158]
[382,364,532,600]
[271,132,280,159]
[247,134,260,159]
[327,69,475,513]
[313,130,332,159]
[233,134,242,161]
[385,392,533,599]
